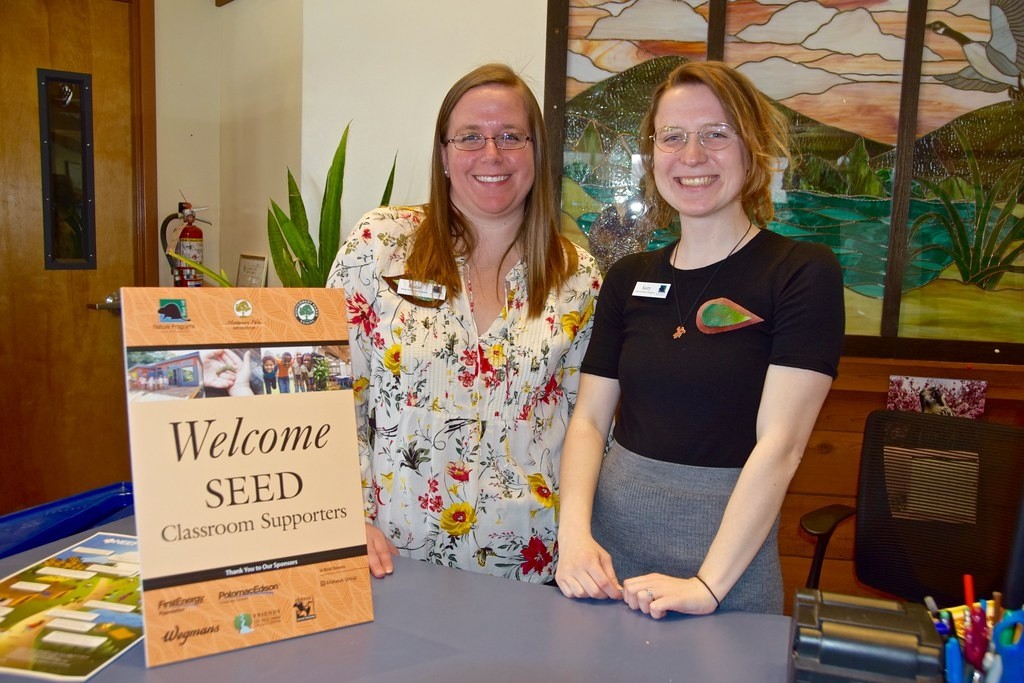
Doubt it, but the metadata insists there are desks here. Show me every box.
[0,517,795,683]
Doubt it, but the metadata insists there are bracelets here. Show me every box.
[695,575,720,608]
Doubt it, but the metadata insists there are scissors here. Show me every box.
[993,610,1024,683]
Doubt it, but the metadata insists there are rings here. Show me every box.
[645,589,655,601]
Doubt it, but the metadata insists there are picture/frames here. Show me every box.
[235,254,268,288]
[542,1,1024,366]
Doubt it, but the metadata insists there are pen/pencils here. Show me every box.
[924,574,1017,683]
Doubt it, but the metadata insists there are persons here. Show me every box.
[326,62,603,583]
[127,350,317,397]
[556,60,848,619]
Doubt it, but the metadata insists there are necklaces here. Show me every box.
[673,217,752,338]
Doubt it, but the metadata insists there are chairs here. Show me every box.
[799,408,1024,611]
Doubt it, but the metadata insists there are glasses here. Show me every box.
[445,132,534,151]
[648,122,740,153]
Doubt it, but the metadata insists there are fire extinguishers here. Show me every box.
[160,202,213,287]
